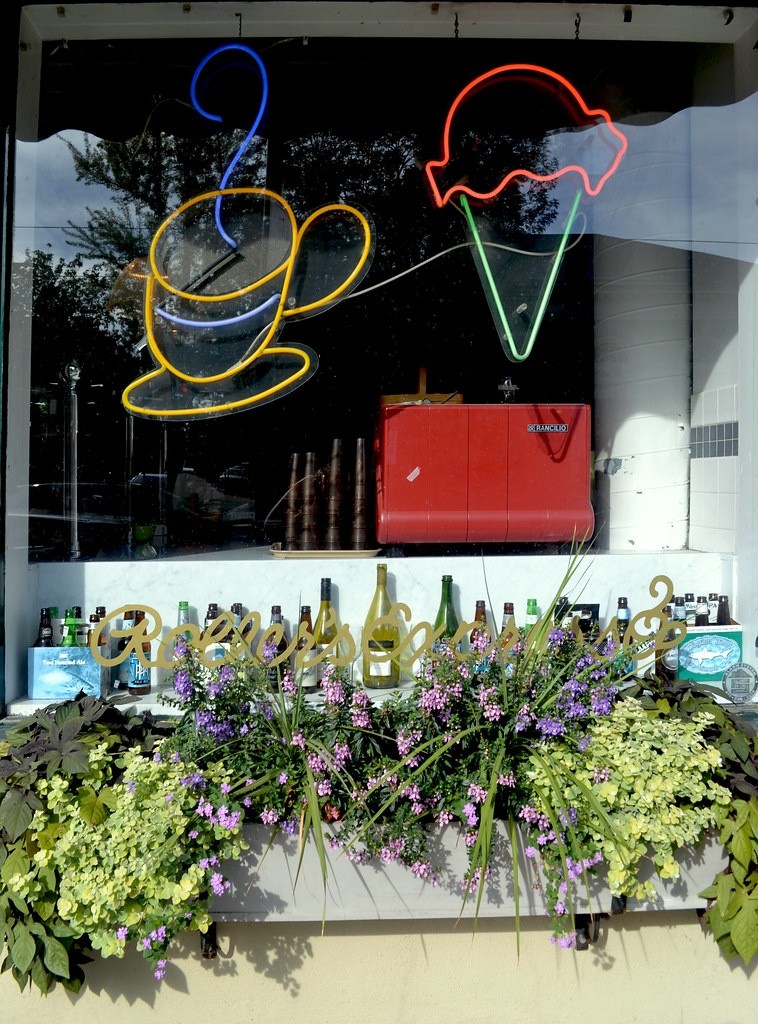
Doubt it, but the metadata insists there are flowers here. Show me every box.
[1,607,758,1003]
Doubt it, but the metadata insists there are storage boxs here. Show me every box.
[672,616,743,682]
[25,644,111,702]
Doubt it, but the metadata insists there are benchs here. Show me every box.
[374,402,595,545]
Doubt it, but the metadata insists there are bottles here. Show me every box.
[264,606,290,693]
[201,601,250,645]
[31,605,108,648]
[433,575,462,664]
[173,601,194,644]
[127,608,151,695]
[654,594,732,683]
[615,597,634,679]
[294,605,318,694]
[311,578,341,686]
[470,599,540,652]
[118,606,137,690]
[362,563,401,688]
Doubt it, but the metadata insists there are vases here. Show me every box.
[174,815,736,958]
